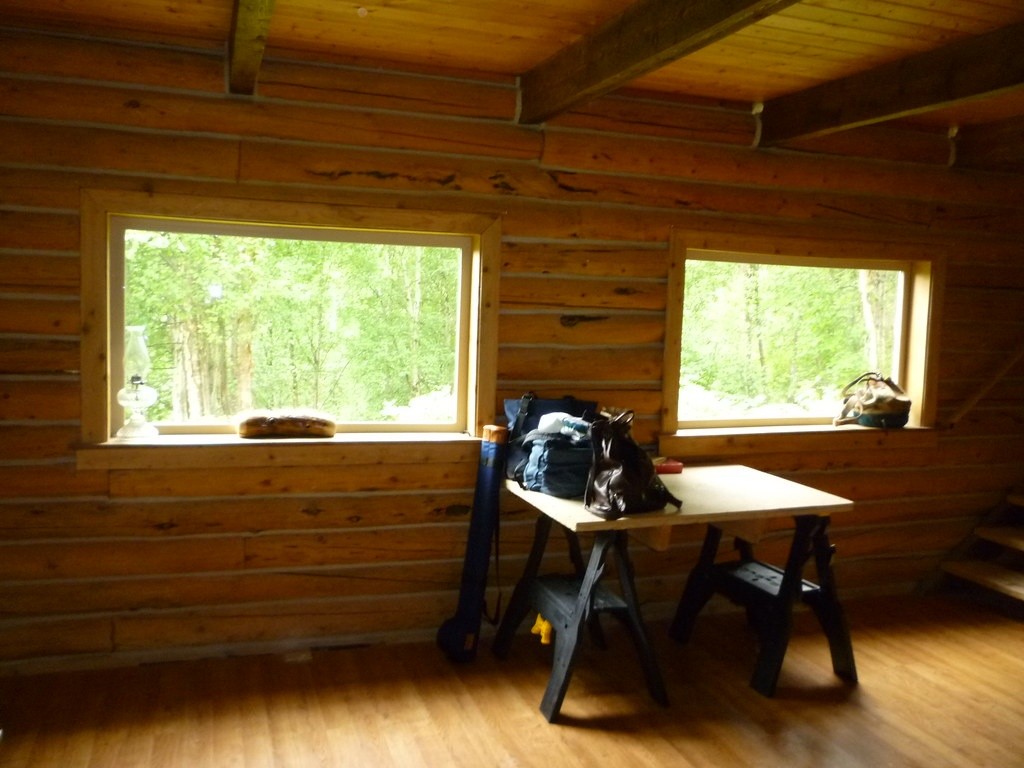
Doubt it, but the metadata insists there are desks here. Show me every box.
[491,464,855,722]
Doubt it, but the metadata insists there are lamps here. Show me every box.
[117,325,161,442]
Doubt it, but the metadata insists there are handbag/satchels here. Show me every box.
[503,390,683,519]
[834,372,912,428]
